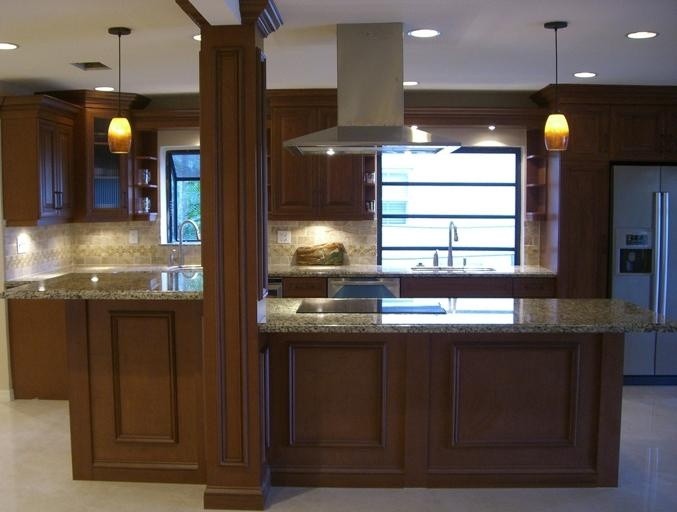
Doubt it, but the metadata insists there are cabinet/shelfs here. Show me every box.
[540,101,675,297]
[283,278,327,297]
[77,89,129,223]
[399,277,512,298]
[1,93,75,227]
[525,121,548,221]
[127,97,159,220]
[270,96,374,221]
[513,278,556,298]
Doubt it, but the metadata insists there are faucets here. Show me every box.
[448,222,459,266]
[179,220,202,266]
[181,271,199,280]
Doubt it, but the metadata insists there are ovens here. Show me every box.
[326,278,401,300]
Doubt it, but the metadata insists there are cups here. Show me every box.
[137,169,150,185]
[135,196,151,214]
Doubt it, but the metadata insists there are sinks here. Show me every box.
[168,266,203,273]
[409,266,496,272]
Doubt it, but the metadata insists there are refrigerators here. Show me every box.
[609,164,677,388]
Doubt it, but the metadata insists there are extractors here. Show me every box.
[279,20,461,157]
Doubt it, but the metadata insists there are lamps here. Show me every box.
[543,22,570,150]
[106,26,133,154]
